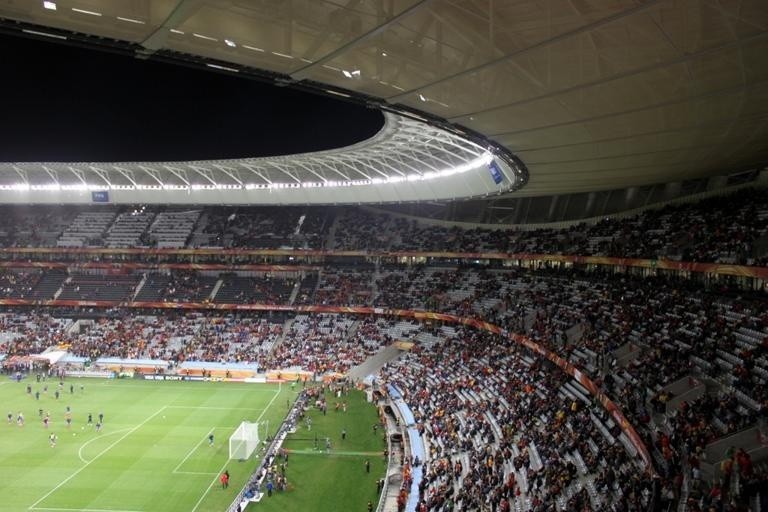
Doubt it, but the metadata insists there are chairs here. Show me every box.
[0,188,766,511]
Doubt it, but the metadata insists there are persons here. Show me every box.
[0,205,768,511]
